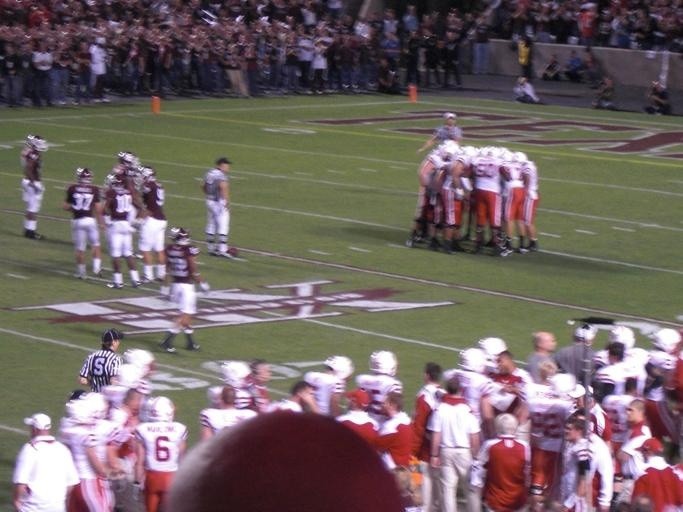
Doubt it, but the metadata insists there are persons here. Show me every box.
[0,0,496,113]
[405,114,541,256]
[592,79,617,109]
[199,350,413,509]
[582,48,606,88]
[411,322,682,511]
[18,133,48,240]
[642,80,672,115]
[54,327,190,509]
[163,407,406,510]
[513,77,544,104]
[201,156,239,259]
[541,54,562,82]
[515,32,535,80]
[562,50,586,82]
[497,2,681,53]
[61,150,210,352]
[13,413,80,509]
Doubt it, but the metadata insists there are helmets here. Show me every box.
[169,225,190,248]
[653,328,680,352]
[479,336,506,357]
[74,167,93,186]
[368,350,396,375]
[223,360,253,390]
[325,355,352,378]
[102,151,154,192]
[495,413,518,440]
[608,326,634,349]
[62,349,155,425]
[148,397,174,422]
[428,141,528,165]
[548,373,575,396]
[24,133,47,153]
[460,348,487,373]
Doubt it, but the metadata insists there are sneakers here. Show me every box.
[406,231,537,256]
[160,344,176,353]
[22,230,44,240]
[69,254,165,289]
[219,252,232,258]
[207,250,220,255]
[187,344,201,351]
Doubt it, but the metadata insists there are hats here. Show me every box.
[22,412,51,431]
[101,327,125,341]
[568,384,595,399]
[215,157,231,167]
[573,322,599,343]
[633,438,663,456]
[443,112,456,119]
[340,386,372,409]
